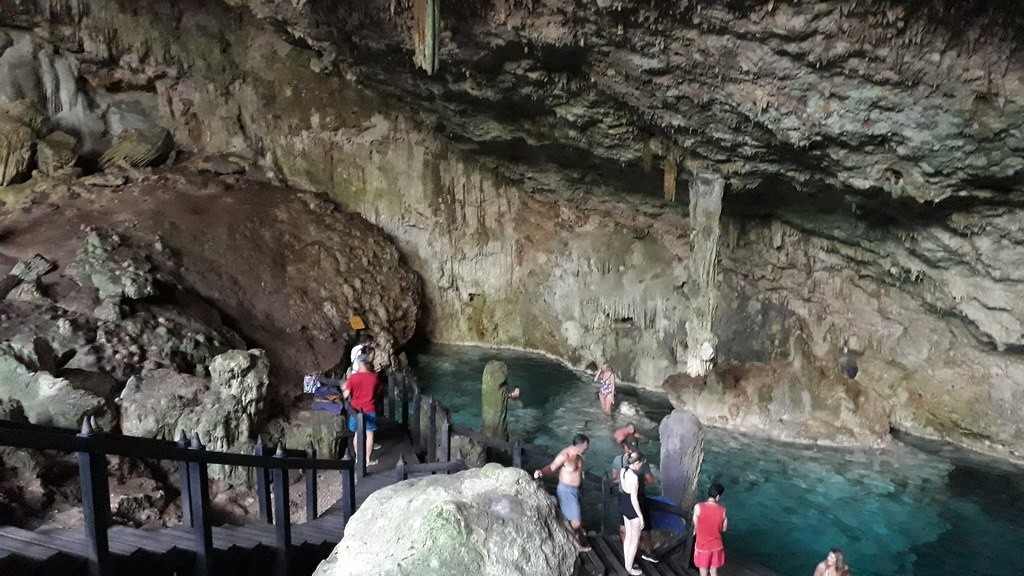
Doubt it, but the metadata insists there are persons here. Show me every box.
[340,334,384,467]
[692,484,728,576]
[813,548,852,576]
[534,423,660,576]
[508,386,521,399]
[594,363,615,416]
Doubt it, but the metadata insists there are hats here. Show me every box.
[623,435,640,452]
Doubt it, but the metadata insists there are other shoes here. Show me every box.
[641,552,659,563]
[372,443,382,450]
